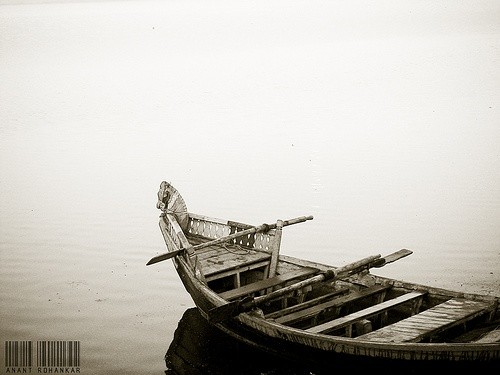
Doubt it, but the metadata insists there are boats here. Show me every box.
[156,181,500,371]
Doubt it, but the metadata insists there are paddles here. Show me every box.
[294,248,413,296]
[146,215,314,266]
[205,253,382,324]
